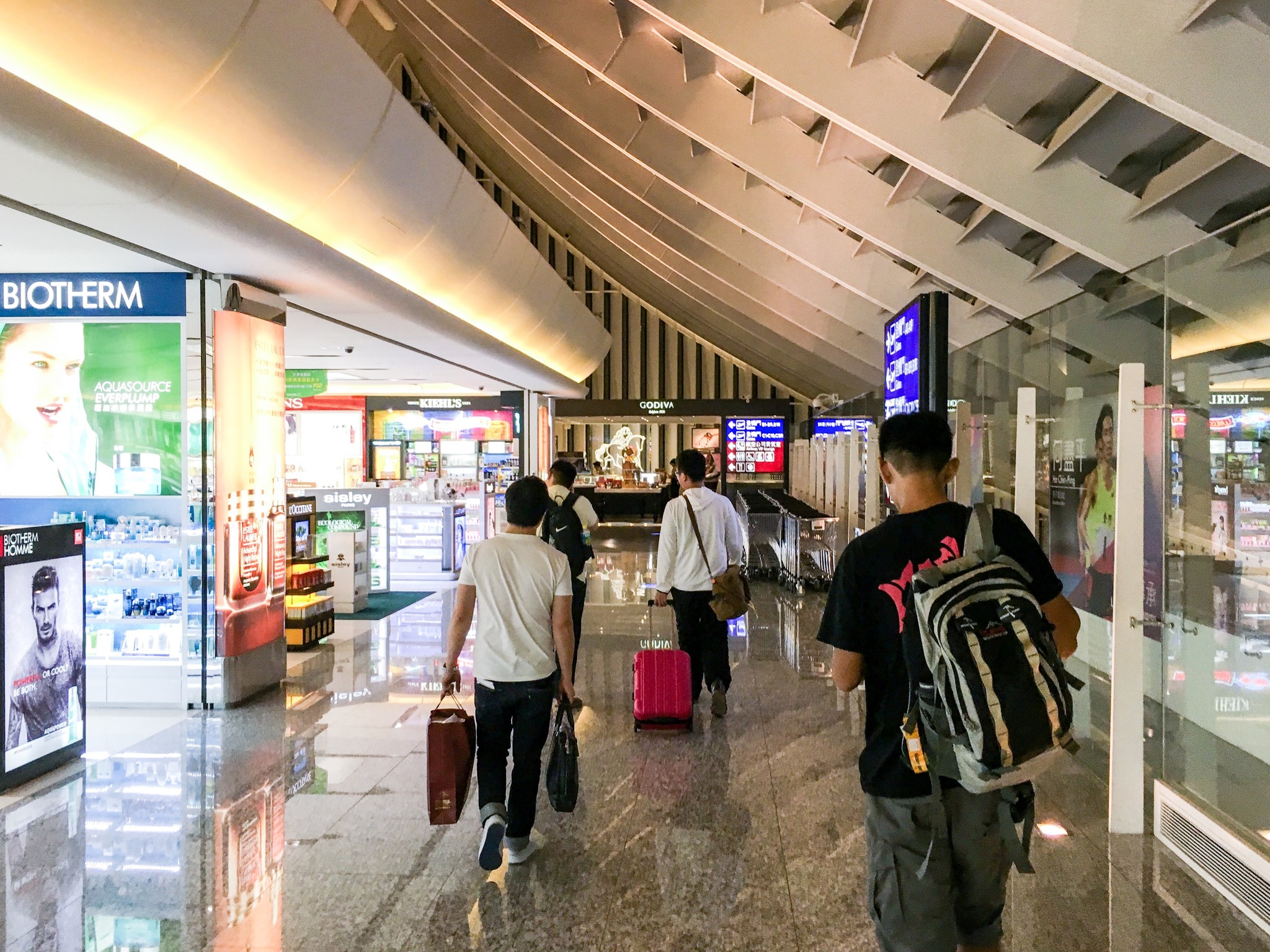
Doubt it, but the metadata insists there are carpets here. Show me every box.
[334,592,436,621]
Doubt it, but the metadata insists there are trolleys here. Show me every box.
[736,488,839,596]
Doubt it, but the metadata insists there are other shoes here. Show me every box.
[478,814,506,871]
[507,826,540,864]
[570,698,583,709]
[710,684,727,718]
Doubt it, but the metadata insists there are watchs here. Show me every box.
[443,663,459,671]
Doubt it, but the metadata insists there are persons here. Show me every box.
[655,715,752,952]
[1076,403,1117,618]
[592,461,604,475]
[536,460,598,722]
[1212,516,1226,555]
[816,408,1082,952]
[441,475,576,871]
[285,415,298,472]
[0,321,116,498]
[663,458,680,499]
[654,449,743,715]
[6,564,85,751]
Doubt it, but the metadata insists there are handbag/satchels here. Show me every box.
[708,565,748,621]
[426,690,476,826]
[545,695,579,812]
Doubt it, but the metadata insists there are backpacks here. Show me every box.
[903,503,1084,795]
[540,491,595,577]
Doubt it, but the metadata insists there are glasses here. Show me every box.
[675,471,687,476]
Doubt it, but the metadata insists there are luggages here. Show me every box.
[632,599,693,733]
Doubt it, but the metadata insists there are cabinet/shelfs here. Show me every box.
[328,529,372,614]
[386,451,514,595]
[188,500,224,710]
[286,554,334,651]
[86,539,181,708]
